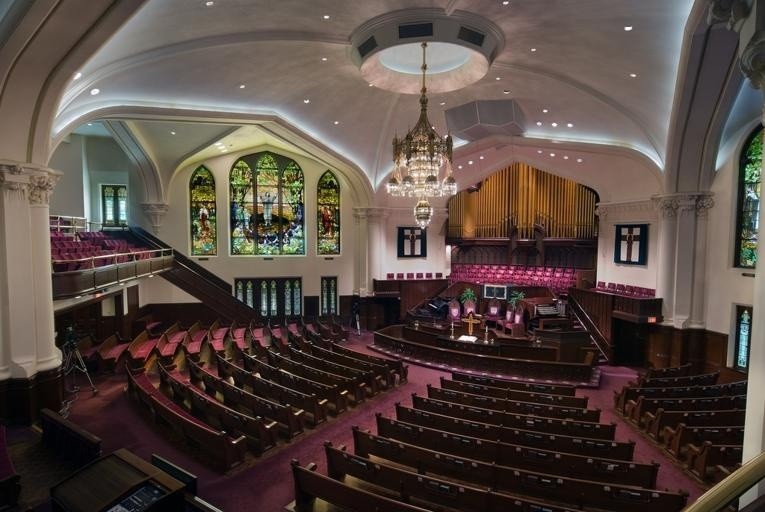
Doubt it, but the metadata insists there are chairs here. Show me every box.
[447,263,574,290]
[51,217,156,269]
[448,297,527,337]
[590,281,656,300]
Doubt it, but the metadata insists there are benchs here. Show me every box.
[94,309,411,468]
[290,360,747,511]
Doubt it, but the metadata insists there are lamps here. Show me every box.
[383,43,458,233]
